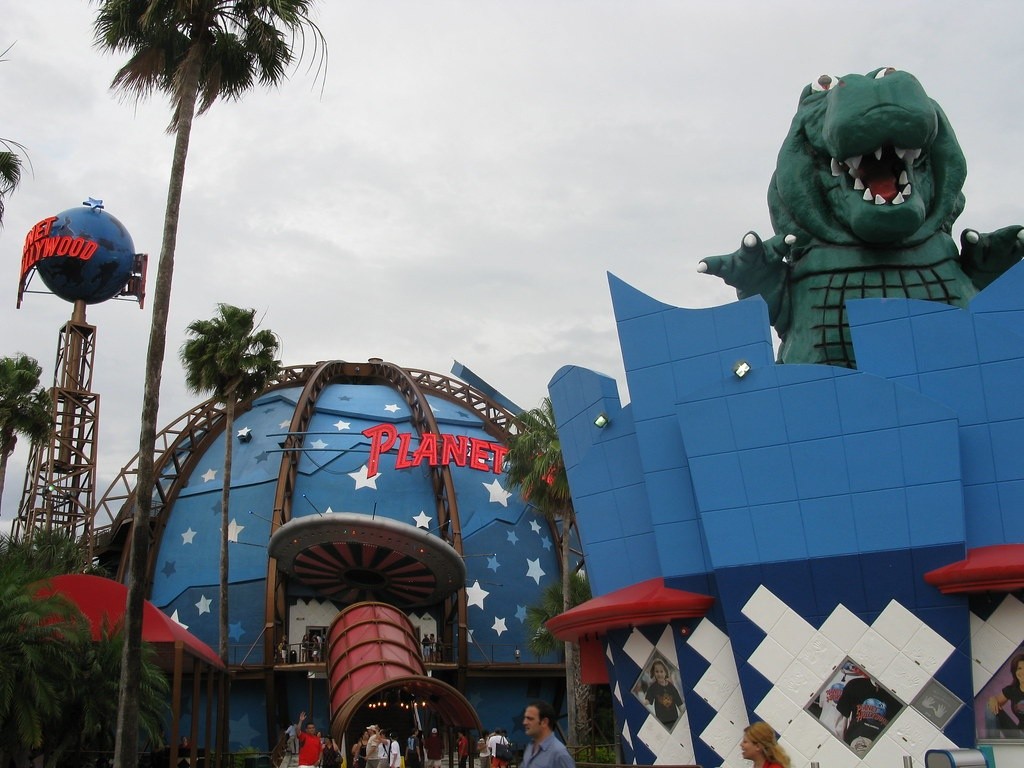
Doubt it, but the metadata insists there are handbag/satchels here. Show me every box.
[334,753,343,764]
[496,736,510,760]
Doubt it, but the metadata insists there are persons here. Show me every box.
[740,722,791,768]
[353,725,401,768]
[521,704,575,768]
[834,667,904,754]
[286,710,343,768]
[405,728,444,768]
[456,730,468,768]
[643,658,685,730]
[281,635,287,664]
[422,633,435,662]
[301,635,322,662]
[477,729,511,768]
[989,654,1024,739]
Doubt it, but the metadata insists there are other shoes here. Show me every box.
[292,753,295,755]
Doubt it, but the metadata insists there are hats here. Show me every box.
[366,725,376,731]
[432,728,438,733]
[390,732,398,737]
